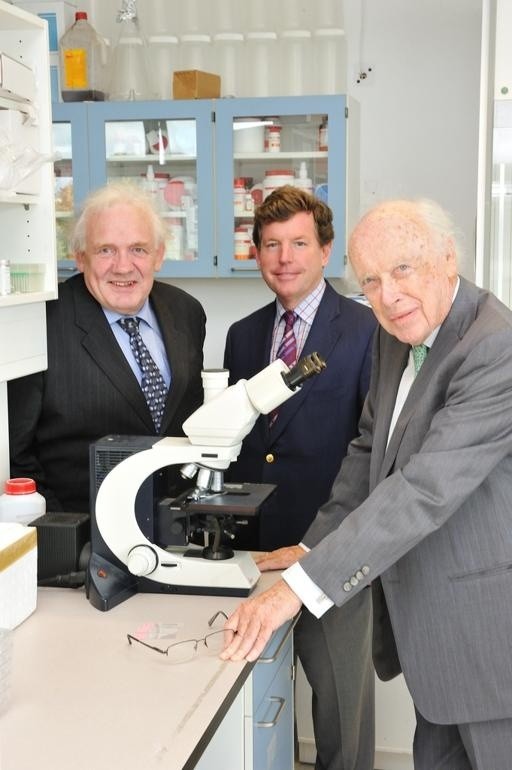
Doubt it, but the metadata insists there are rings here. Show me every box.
[266,552,269,558]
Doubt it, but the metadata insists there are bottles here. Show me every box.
[138,114,328,262]
[106,0,152,102]
[0,478,46,527]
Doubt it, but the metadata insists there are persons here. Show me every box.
[225,185,379,768]
[9,183,208,513]
[218,197,511,770]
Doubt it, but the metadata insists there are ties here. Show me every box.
[268,309,299,426]
[411,342,429,383]
[117,315,169,435]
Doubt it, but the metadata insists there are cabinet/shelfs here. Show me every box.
[51,101,213,280]
[243,599,306,769]
[0,1,59,309]
[213,95,358,280]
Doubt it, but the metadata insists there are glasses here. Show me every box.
[125,609,240,663]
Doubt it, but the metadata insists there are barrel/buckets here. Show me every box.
[58,11,108,100]
